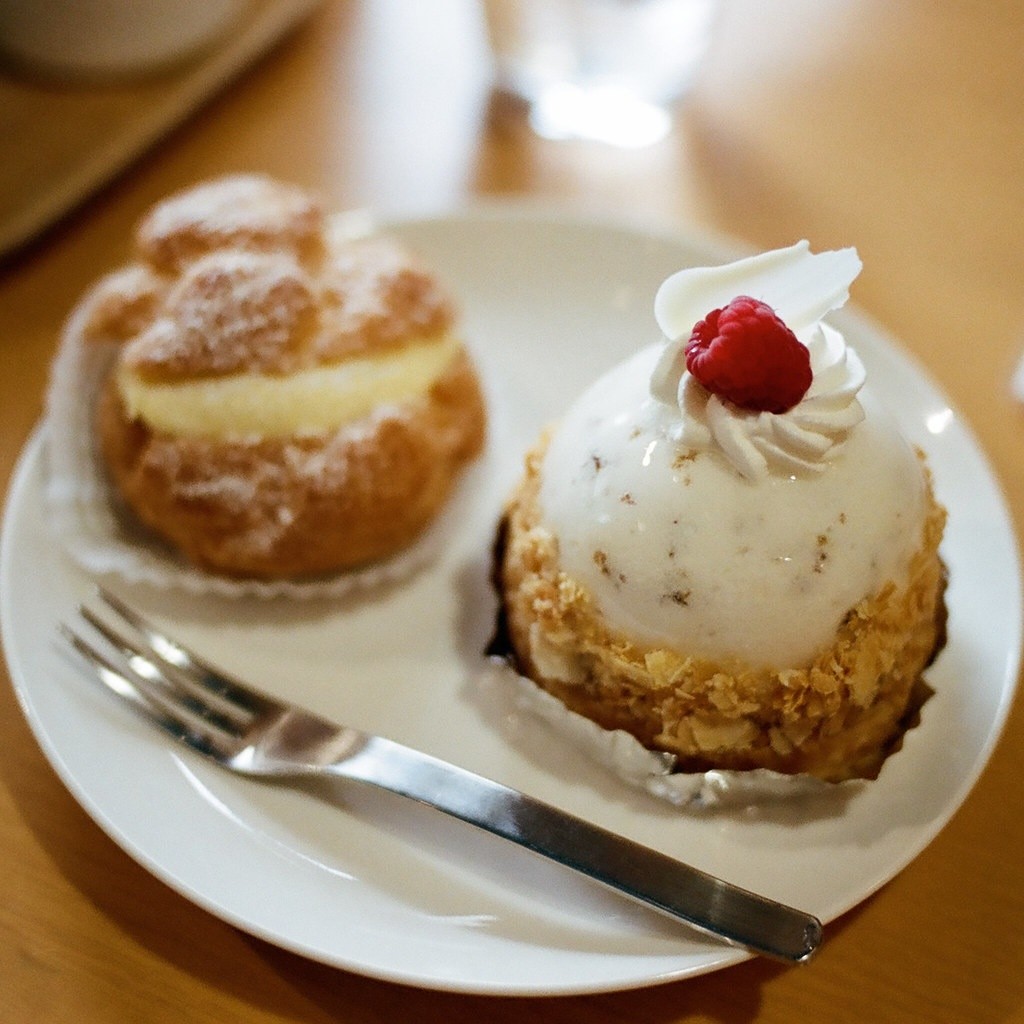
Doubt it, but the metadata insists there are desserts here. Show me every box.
[476,238,953,802]
[38,173,484,599]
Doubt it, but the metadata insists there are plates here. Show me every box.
[0,198,1023,996]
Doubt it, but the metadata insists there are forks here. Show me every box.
[57,585,829,966]
